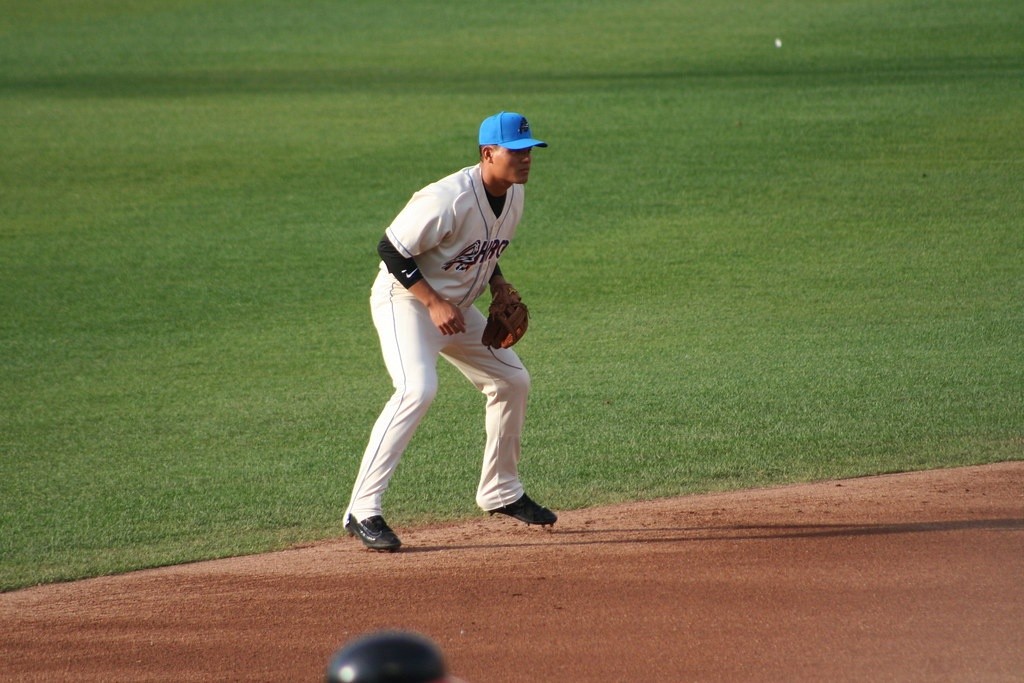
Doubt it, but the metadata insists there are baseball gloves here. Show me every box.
[481,282,529,350]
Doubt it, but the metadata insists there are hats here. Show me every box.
[478,111,548,151]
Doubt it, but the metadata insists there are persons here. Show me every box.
[342,111,558,553]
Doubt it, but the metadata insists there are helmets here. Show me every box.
[327,632,445,683]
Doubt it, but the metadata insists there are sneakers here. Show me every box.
[345,514,401,551]
[489,493,557,528]
